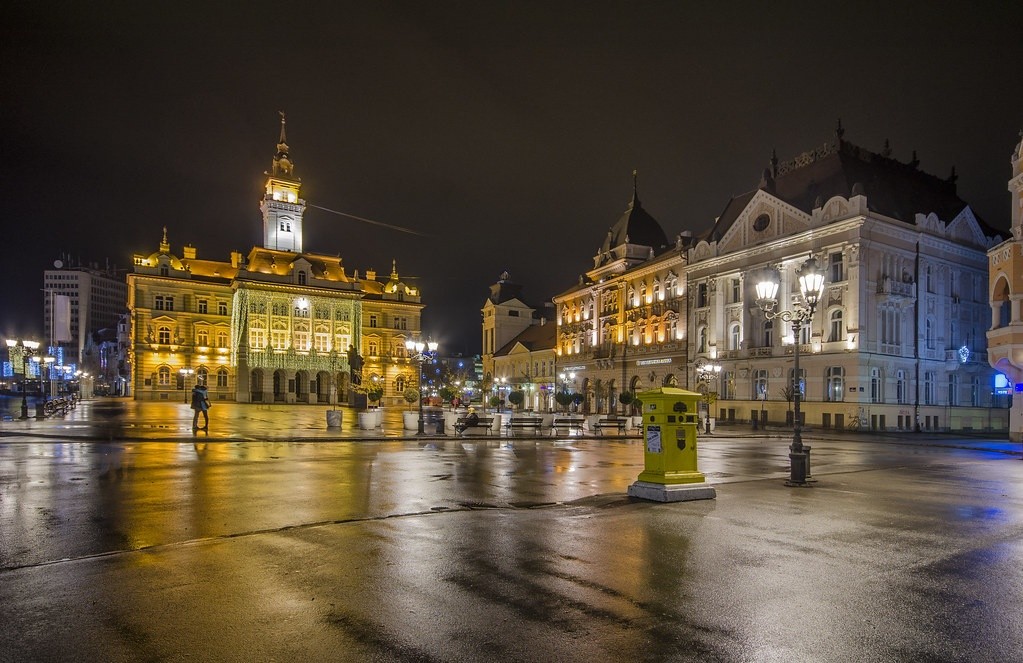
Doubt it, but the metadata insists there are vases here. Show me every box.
[524,415,537,431]
[588,414,602,431]
[597,414,609,428]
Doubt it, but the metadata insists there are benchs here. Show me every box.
[452,418,494,437]
[505,417,544,436]
[553,418,586,434]
[593,418,628,435]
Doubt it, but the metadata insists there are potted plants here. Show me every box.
[571,393,585,428]
[484,396,501,431]
[555,392,573,430]
[701,392,719,432]
[631,399,644,427]
[508,391,523,429]
[401,388,419,430]
[617,390,634,430]
[367,387,383,427]
[438,385,464,430]
[349,369,375,431]
[539,393,555,427]
[325,373,348,427]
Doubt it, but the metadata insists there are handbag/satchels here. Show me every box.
[204,397,211,408]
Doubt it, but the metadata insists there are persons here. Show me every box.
[190,384,212,432]
[346,344,364,385]
[453,407,478,434]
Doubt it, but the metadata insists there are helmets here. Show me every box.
[467,408,475,413]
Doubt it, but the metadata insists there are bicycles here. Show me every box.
[44,394,80,414]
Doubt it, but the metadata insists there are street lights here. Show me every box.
[494,374,507,412]
[404,332,438,434]
[696,358,724,435]
[32,355,95,409]
[5,335,41,419]
[559,371,576,413]
[755,250,828,486]
[181,367,195,403]
